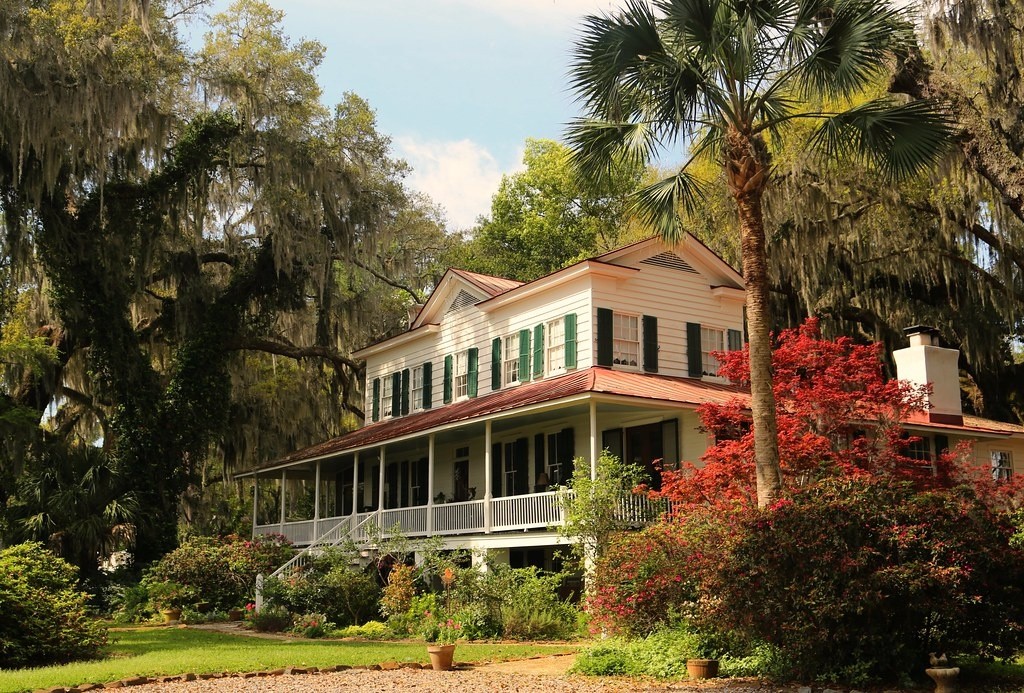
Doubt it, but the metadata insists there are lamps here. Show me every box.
[538,472,552,490]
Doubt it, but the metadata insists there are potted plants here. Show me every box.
[686,631,719,679]
[229,607,244,621]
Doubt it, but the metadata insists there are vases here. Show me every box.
[427,644,455,671]
[163,609,181,622]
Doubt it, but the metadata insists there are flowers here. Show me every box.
[423,610,463,645]
[161,592,179,611]
